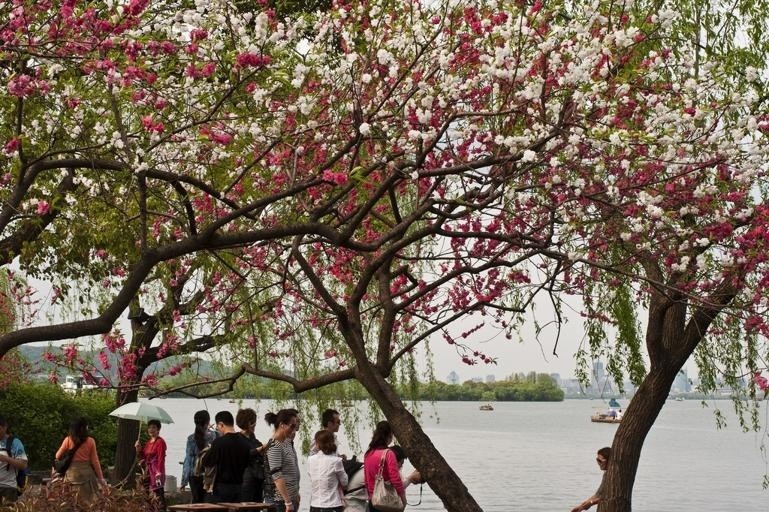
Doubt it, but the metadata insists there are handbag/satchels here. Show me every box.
[372,476,405,512]
[54,450,72,474]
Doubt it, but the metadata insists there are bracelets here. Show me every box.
[591,501,593,505]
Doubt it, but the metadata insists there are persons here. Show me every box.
[572,447,612,512]
[0,416,28,507]
[134,420,167,512]
[56,417,107,503]
[179,408,426,512]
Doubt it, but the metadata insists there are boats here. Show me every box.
[590,398,626,423]
[480,405,494,411]
[60,375,105,395]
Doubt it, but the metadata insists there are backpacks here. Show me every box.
[6,435,28,489]
[340,455,365,495]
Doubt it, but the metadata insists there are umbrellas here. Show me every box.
[109,401,174,440]
